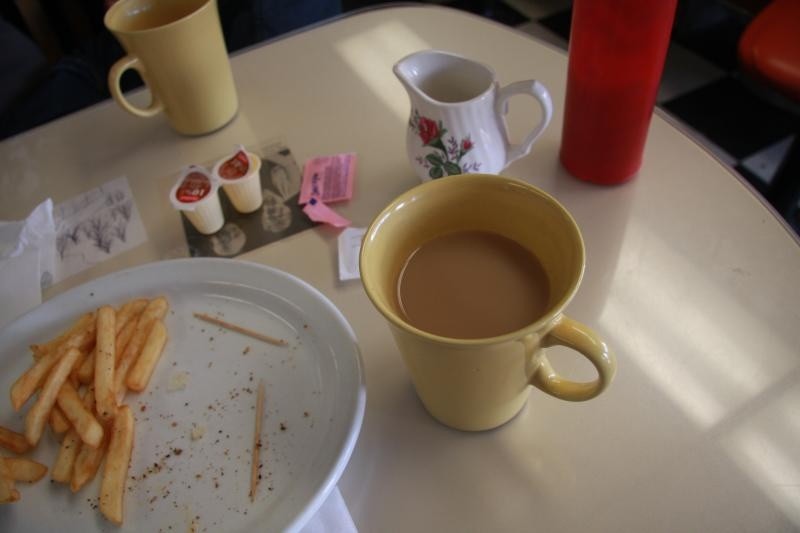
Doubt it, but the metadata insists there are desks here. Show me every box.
[1,3,800,532]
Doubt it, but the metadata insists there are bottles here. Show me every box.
[559,0,678,185]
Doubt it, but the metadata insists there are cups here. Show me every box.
[359,173,615,432]
[213,152,263,213]
[170,165,222,235]
[103,0,238,136]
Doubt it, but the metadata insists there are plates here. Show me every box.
[0,258,368,533]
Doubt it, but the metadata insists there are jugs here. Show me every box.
[393,49,553,184]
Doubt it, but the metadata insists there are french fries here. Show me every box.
[0,295,170,525]
